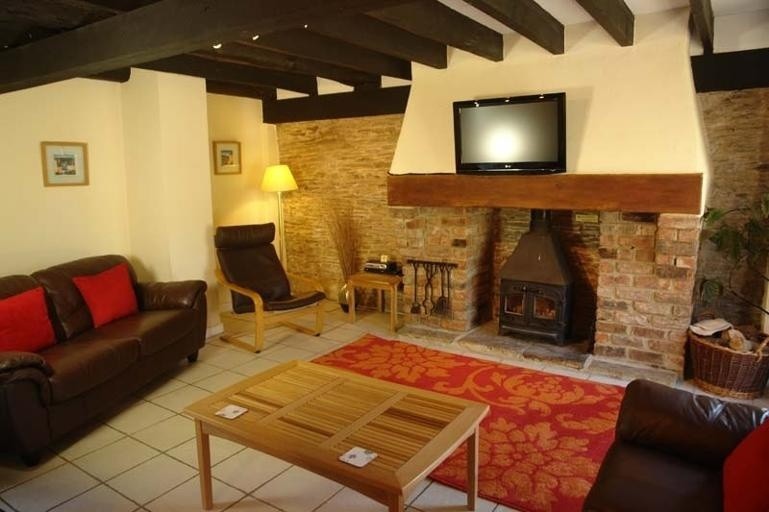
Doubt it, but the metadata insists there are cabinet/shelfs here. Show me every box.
[499,278,570,343]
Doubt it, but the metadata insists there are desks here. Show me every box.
[348,271,404,334]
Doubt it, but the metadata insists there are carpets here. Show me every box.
[309,335,629,510]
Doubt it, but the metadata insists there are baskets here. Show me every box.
[688,312,768,399]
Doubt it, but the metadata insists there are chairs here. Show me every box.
[212,222,326,354]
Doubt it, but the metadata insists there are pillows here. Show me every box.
[721,416,767,512]
[0,285,57,354]
[72,263,146,330]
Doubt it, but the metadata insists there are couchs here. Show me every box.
[582,378,768,512]
[0,253,209,472]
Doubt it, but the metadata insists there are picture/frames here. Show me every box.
[212,138,243,176]
[39,140,91,187]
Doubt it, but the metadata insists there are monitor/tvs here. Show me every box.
[453,92,566,175]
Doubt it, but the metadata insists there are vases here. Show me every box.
[338,281,359,314]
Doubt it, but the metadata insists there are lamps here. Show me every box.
[259,163,298,276]
